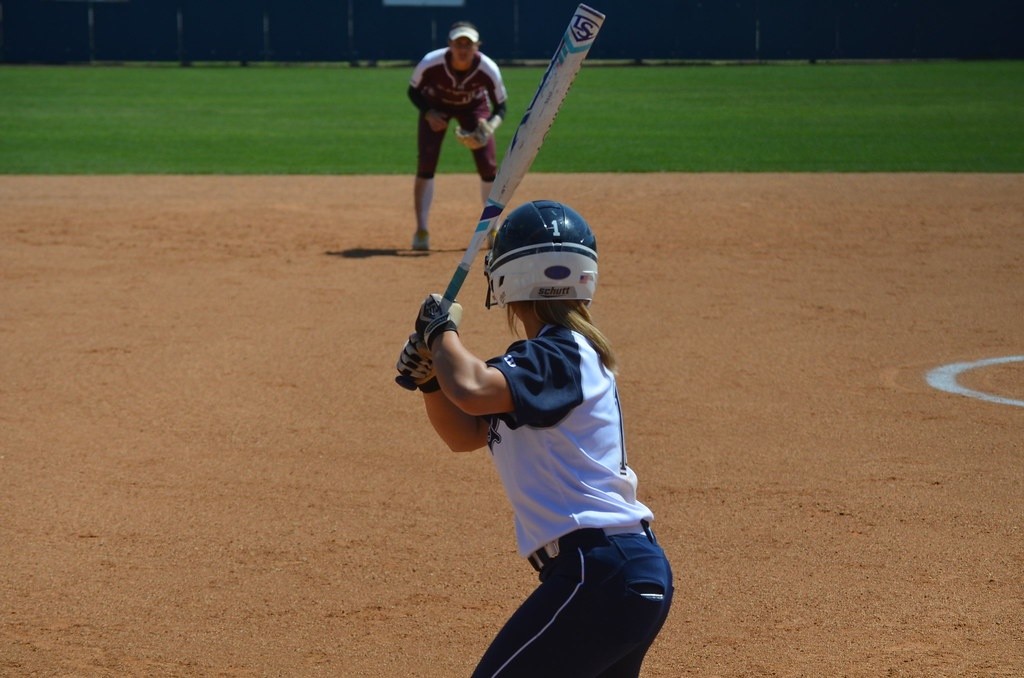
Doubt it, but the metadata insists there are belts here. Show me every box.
[527,522,647,573]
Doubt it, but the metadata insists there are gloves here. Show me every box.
[396,331,441,394]
[414,293,463,351]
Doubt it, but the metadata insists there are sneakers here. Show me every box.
[487,228,498,249]
[412,228,431,251]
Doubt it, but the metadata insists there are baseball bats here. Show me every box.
[393,3,608,393]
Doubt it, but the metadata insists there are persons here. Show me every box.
[397,200,674,678]
[406,22,508,250]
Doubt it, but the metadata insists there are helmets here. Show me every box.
[483,199,598,310]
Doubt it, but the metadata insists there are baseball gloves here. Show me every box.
[451,117,491,149]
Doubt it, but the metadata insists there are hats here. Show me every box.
[448,20,480,43]
